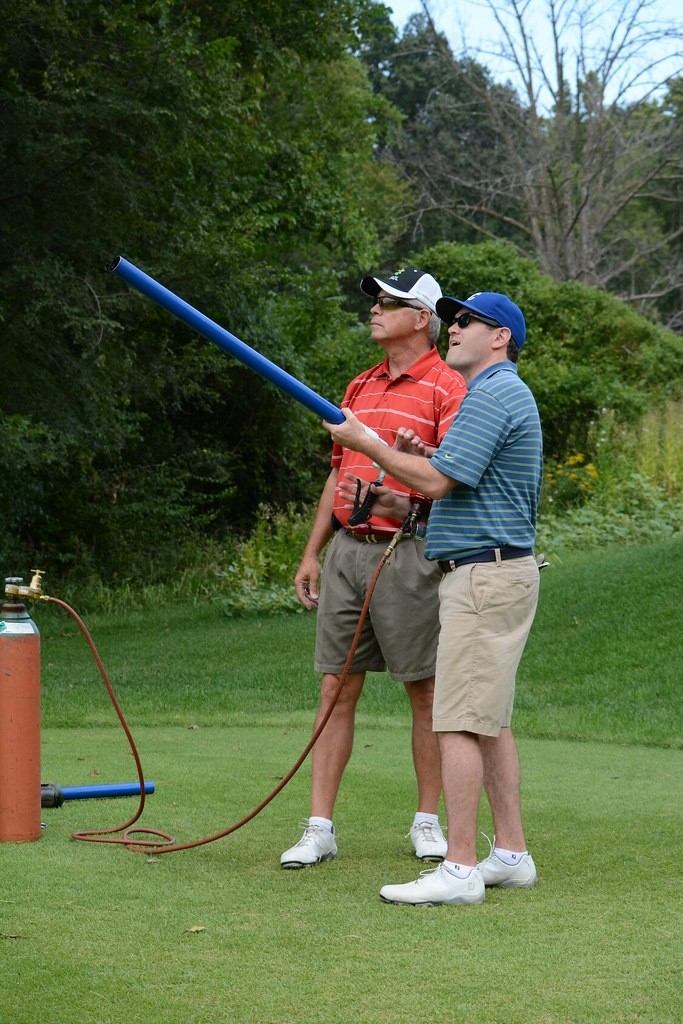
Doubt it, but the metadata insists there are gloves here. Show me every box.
[535,552,551,570]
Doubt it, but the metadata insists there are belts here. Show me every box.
[341,525,396,544]
[436,547,534,573]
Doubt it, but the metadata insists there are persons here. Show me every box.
[321,291,543,907]
[279,267,469,870]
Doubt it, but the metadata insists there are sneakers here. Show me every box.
[477,831,537,888]
[379,862,486,906]
[278,817,338,869]
[404,820,448,861]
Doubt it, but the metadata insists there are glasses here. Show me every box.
[372,295,433,317]
[447,311,501,329]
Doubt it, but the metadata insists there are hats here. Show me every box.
[359,267,443,319]
[434,291,527,350]
